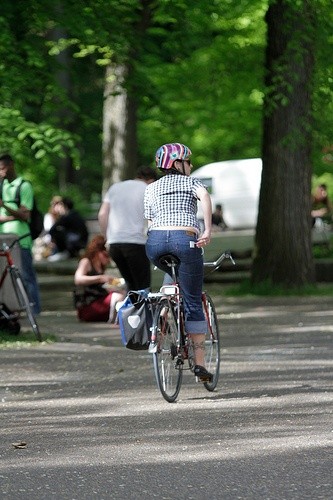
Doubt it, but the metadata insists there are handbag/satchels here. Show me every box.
[118,287,153,350]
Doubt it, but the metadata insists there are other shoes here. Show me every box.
[160,316,170,334]
[194,365,214,381]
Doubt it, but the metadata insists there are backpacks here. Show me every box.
[0,180,43,240]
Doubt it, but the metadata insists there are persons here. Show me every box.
[74,235,128,323]
[98,165,156,303]
[211,205,228,231]
[309,184,331,232]
[0,154,42,316]
[144,143,213,380]
[36,196,88,263]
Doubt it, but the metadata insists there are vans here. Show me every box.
[189,157,264,231]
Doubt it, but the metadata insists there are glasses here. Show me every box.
[178,160,190,166]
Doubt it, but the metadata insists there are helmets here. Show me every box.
[155,143,192,169]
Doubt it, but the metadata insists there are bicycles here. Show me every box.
[0,234,42,339]
[145,250,235,403]
